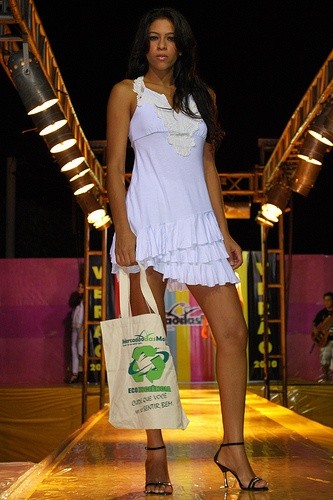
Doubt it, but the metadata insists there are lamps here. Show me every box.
[307,94,333,147]
[290,159,322,197]
[76,193,111,229]
[44,123,78,153]
[257,171,291,228]
[55,144,86,173]
[63,161,91,183]
[71,174,94,196]
[32,103,68,136]
[0,30,60,116]
[297,132,327,166]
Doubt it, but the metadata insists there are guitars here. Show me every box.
[311,315,333,347]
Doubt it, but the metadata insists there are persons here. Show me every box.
[312,292,333,383]
[68,281,85,383]
[105,8,268,496]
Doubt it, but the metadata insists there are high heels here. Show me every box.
[144,446,173,495]
[67,375,78,384]
[214,442,268,491]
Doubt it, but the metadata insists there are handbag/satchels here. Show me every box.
[100,262,185,429]
